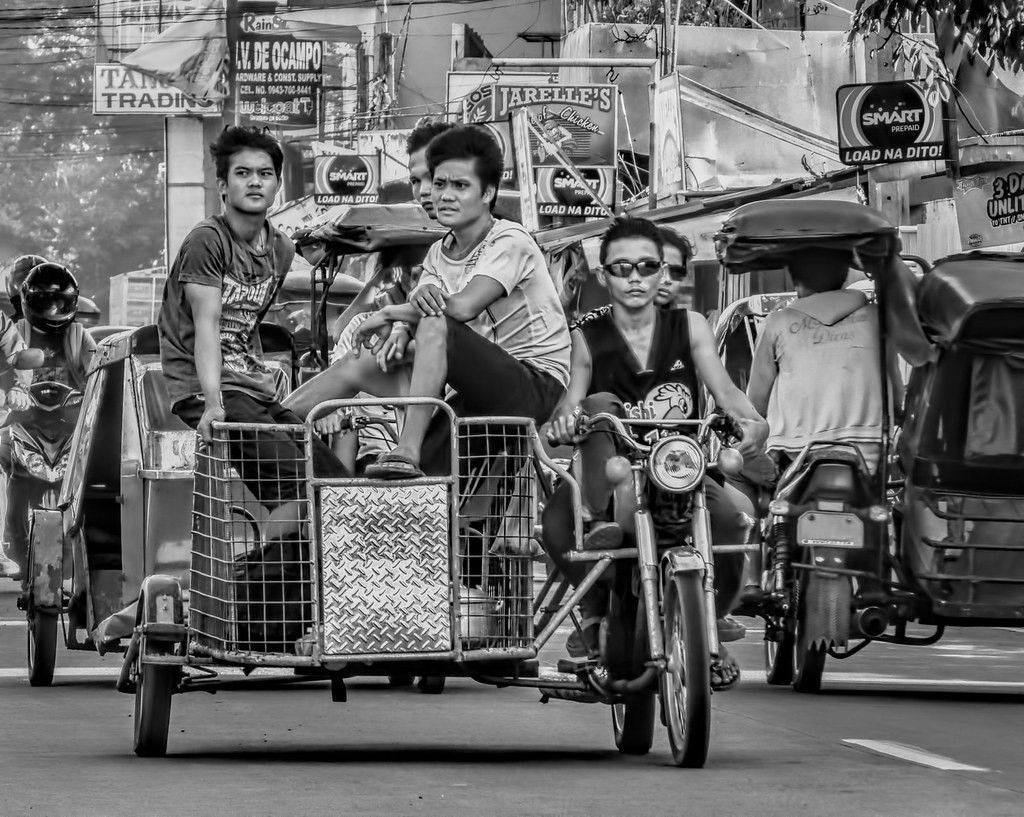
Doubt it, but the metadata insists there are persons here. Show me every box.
[0,255,100,578]
[279,122,571,478]
[541,216,769,694]
[730,247,904,618]
[156,125,354,512]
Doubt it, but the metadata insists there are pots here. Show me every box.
[447,581,505,643]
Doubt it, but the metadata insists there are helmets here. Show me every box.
[5,255,48,304]
[20,263,79,333]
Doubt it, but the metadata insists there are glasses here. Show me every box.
[598,261,667,278]
[669,264,687,281]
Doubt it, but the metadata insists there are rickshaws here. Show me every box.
[0,321,762,770]
[699,196,1024,694]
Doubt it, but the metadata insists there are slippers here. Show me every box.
[585,523,624,580]
[710,643,739,691]
[365,454,426,479]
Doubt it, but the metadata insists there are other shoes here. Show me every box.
[740,584,763,605]
[566,617,600,656]
[715,616,745,640]
[863,582,887,602]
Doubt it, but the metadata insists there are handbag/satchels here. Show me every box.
[230,506,312,648]
